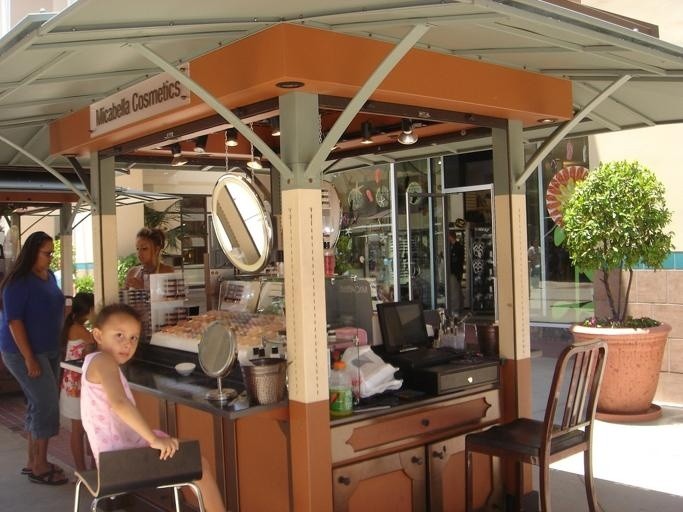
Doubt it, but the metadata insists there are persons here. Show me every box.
[529,238,541,288]
[122,225,174,291]
[0,233,69,486]
[448,231,465,312]
[60,291,97,484]
[78,302,227,512]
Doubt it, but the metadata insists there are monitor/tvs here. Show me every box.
[376,302,429,353]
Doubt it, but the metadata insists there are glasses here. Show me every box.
[40,249,54,257]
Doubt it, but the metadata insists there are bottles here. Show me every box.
[252,347,279,361]
[323,240,334,275]
[327,323,338,344]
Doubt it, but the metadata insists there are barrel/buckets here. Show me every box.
[478,323,499,360]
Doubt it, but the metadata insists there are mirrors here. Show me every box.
[210,170,275,275]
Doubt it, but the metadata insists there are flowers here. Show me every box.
[581,313,658,328]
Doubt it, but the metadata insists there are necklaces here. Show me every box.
[140,267,156,280]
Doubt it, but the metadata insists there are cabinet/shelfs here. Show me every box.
[329,378,509,512]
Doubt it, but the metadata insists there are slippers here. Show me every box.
[21,461,63,473]
[29,473,68,485]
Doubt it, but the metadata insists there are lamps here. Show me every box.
[321,116,420,151]
[169,114,282,174]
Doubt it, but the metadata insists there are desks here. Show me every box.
[58,356,288,420]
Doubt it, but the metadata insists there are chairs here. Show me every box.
[70,438,205,512]
[464,338,610,512]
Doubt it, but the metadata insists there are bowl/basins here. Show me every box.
[173,362,195,377]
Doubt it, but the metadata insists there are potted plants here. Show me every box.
[560,158,676,415]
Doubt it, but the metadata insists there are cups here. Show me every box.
[181,248,190,265]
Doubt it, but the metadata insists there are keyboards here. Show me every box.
[390,347,467,367]
